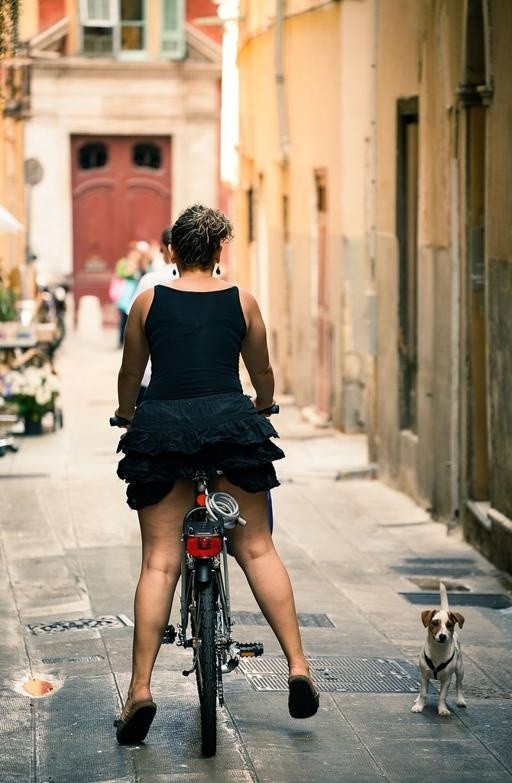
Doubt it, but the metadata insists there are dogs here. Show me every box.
[409,607,468,718]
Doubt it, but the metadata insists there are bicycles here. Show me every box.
[105,404,280,759]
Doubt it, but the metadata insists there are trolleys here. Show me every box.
[0,315,69,438]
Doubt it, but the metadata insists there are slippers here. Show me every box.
[114,699,156,745]
[288,675,319,719]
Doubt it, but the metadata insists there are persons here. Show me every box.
[37,267,71,377]
[113,227,226,352]
[115,202,322,746]
